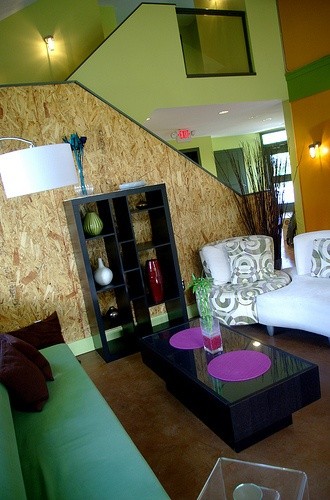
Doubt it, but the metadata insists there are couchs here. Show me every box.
[0,342,174,500]
[252,227,330,340]
[194,233,291,328]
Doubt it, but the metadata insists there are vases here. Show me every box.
[83,211,103,237]
[94,257,115,286]
[146,258,164,303]
[104,305,121,329]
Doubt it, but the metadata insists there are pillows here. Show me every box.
[226,238,277,282]
[311,237,330,278]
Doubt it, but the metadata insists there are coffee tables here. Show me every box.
[135,318,323,455]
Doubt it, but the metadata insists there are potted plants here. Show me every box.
[188,269,224,354]
[61,132,94,197]
[211,134,288,271]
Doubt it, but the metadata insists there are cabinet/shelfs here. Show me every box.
[61,182,190,365]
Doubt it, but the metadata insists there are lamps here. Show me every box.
[43,33,57,51]
[307,140,326,159]
[0,137,82,199]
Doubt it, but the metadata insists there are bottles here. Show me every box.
[145,259,164,303]
[136,200,149,210]
[93,258,114,287]
[82,211,104,237]
[106,305,121,322]
